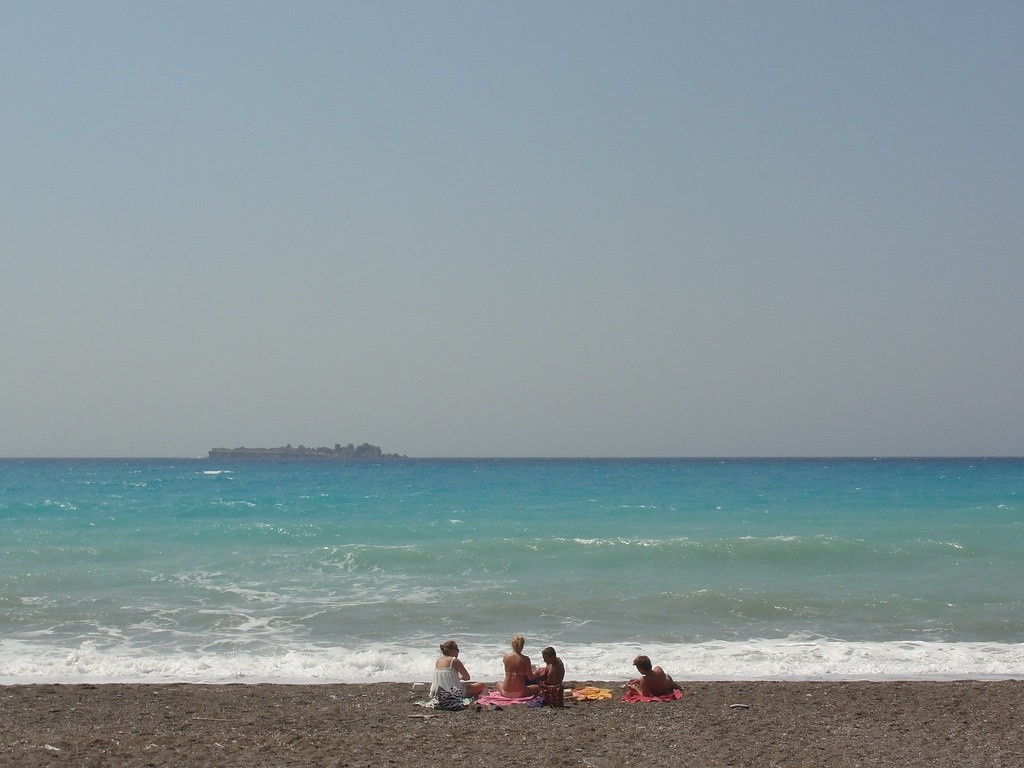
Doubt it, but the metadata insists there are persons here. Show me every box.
[524,646,566,692]
[499,635,541,699]
[427,640,485,704]
[625,654,681,701]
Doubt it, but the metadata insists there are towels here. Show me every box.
[411,684,684,711]
